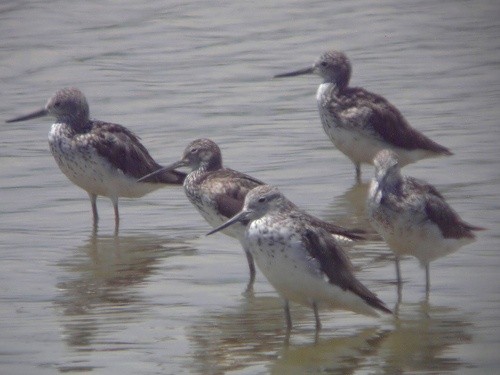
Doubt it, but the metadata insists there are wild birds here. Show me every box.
[272,49,454,183]
[204,183,393,329]
[366,145,485,306]
[4,88,189,236]
[135,137,369,293]
